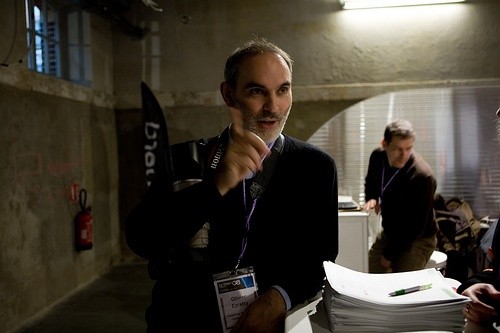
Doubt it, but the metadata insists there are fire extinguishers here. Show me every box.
[73,189,93,252]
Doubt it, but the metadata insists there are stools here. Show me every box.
[424,251,448,276]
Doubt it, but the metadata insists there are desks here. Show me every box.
[285,290,500,333]
[334,210,370,273]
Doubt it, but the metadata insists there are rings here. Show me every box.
[465,304,470,313]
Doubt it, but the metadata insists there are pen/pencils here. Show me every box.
[388,284,432,296]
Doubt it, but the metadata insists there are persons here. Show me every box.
[364,120,438,272]
[136,35,340,333]
[457,217,500,326]
